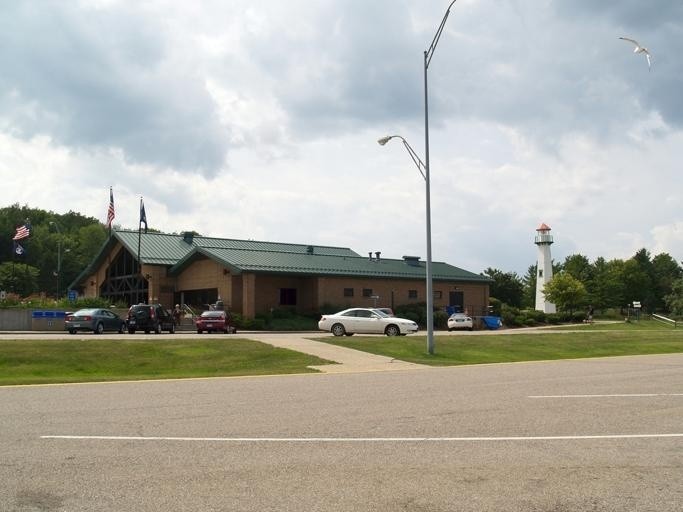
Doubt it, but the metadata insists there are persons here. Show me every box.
[172,304,182,326]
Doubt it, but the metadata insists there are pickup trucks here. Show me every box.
[446,305,502,330]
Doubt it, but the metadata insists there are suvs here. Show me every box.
[124,302,177,335]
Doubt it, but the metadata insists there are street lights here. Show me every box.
[48,221,63,306]
[377,129,444,357]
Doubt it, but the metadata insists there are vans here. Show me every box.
[376,307,394,317]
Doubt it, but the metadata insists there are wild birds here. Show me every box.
[619,37,651,72]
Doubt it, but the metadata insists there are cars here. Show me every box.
[63,308,127,334]
[319,306,420,337]
[195,310,239,336]
[446,312,477,330]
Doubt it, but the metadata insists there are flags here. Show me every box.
[140,202,147,233]
[106,187,115,225]
[15,240,28,258]
[12,222,31,241]
